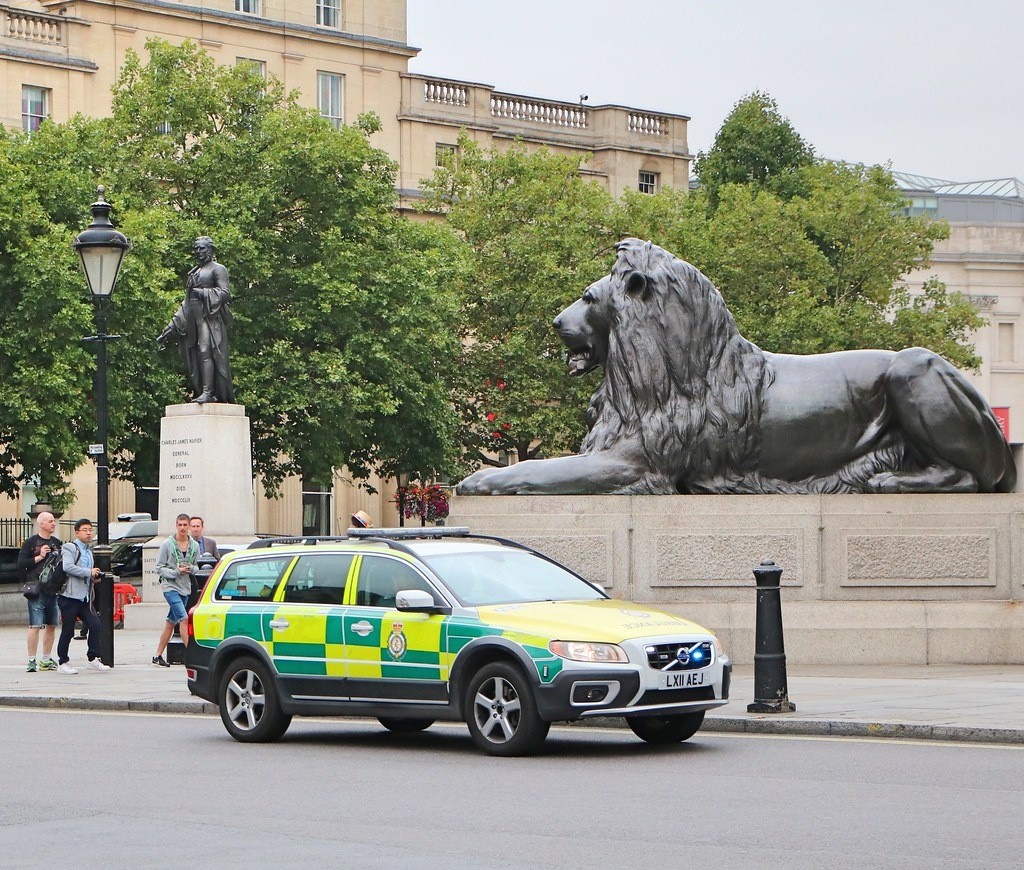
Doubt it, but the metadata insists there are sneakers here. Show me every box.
[86,657,110,671]
[151,655,170,668]
[26,658,38,672]
[39,658,58,671]
[57,661,78,674]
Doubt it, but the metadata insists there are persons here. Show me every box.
[17,512,110,674]
[189,516,220,561]
[152,514,201,667]
[162,236,234,404]
[350,510,375,529]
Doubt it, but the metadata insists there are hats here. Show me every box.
[351,509,374,528]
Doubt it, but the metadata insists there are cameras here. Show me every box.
[95,571,105,580]
[48,544,56,553]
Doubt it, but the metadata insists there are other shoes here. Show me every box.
[73,636,87,640]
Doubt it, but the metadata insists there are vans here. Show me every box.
[87,513,158,552]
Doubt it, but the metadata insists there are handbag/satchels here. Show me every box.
[22,582,41,602]
[38,549,70,595]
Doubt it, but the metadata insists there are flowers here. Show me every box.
[415,484,452,523]
[393,485,426,520]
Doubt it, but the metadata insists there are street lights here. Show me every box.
[73,183,131,670]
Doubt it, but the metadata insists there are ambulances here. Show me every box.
[185,522,734,759]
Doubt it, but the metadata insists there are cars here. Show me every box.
[108,536,154,580]
